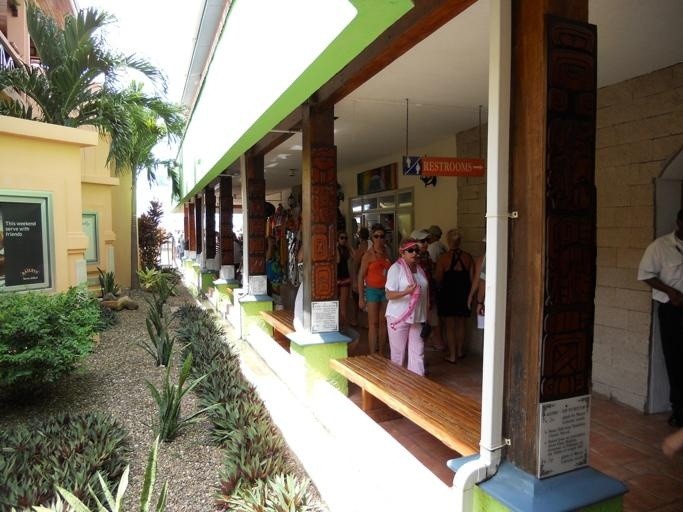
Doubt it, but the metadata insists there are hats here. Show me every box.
[409,229,432,240]
[400,242,422,254]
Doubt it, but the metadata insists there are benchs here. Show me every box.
[261,308,298,340]
[332,351,484,457]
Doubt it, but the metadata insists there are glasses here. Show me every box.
[374,234,385,238]
[339,236,348,240]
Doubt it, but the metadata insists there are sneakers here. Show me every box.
[429,343,464,363]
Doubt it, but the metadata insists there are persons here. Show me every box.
[336,223,486,376]
[636,205,682,429]
[214,232,282,311]
[658,427,683,460]
[173,233,186,261]
